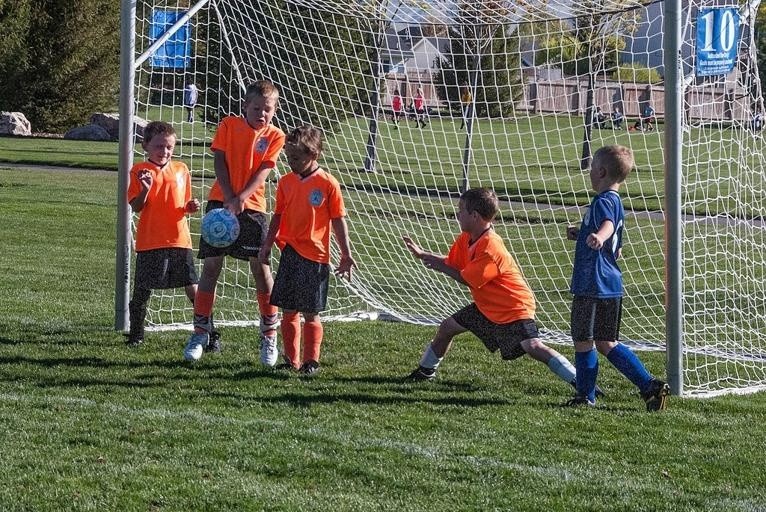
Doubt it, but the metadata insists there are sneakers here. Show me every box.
[259,331,280,369]
[125,332,145,347]
[277,354,319,376]
[398,365,437,383]
[639,374,671,413]
[183,331,211,362]
[206,329,222,355]
[562,384,605,406]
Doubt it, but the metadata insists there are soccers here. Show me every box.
[201,207,239,247]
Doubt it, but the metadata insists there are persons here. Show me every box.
[460,85,472,129]
[593,107,608,129]
[391,90,402,129]
[257,127,359,380]
[642,102,655,132]
[125,121,220,349]
[612,107,624,130]
[560,145,671,412]
[185,79,198,122]
[414,88,427,128]
[184,80,286,366]
[400,187,607,403]
